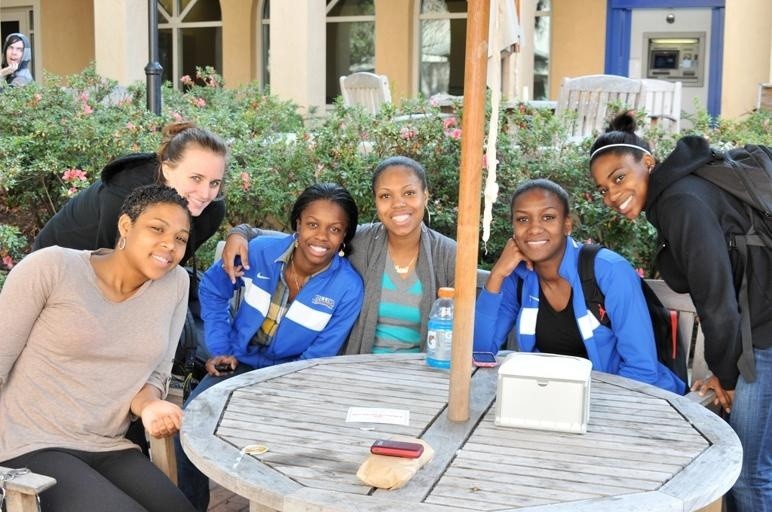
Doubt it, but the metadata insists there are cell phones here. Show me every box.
[369,439,424,460]
[472,352,498,368]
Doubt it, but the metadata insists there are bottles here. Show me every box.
[424,285,455,369]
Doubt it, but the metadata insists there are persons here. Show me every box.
[22,117,233,511]
[474,176,689,395]
[586,107,771,512]
[174,179,363,511]
[1,32,38,92]
[2,182,198,511]
[220,152,454,357]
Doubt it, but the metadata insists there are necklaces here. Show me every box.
[383,242,419,274]
[290,257,302,297]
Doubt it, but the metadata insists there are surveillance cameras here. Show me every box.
[666,13,675,24]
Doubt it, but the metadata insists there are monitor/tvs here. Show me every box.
[651,50,678,71]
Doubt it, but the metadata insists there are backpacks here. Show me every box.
[514,242,688,387]
[652,141,771,252]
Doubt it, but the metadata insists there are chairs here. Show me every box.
[634,75,683,135]
[551,71,646,150]
[0,423,180,512]
[336,70,434,120]
[642,275,726,415]
[473,268,522,353]
[213,241,246,318]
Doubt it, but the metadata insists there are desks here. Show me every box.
[180,353,748,511]
[431,96,559,117]
